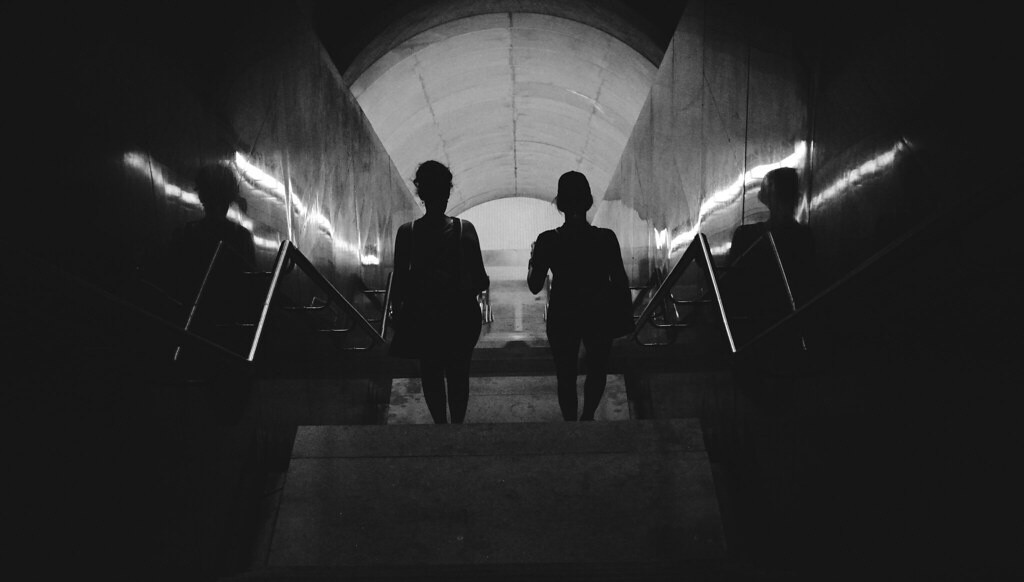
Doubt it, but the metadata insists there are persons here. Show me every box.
[179,164,253,346]
[525,170,633,420]
[724,168,815,346]
[387,158,494,428]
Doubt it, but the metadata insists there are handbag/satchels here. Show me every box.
[389,297,422,358]
[601,276,635,338]
